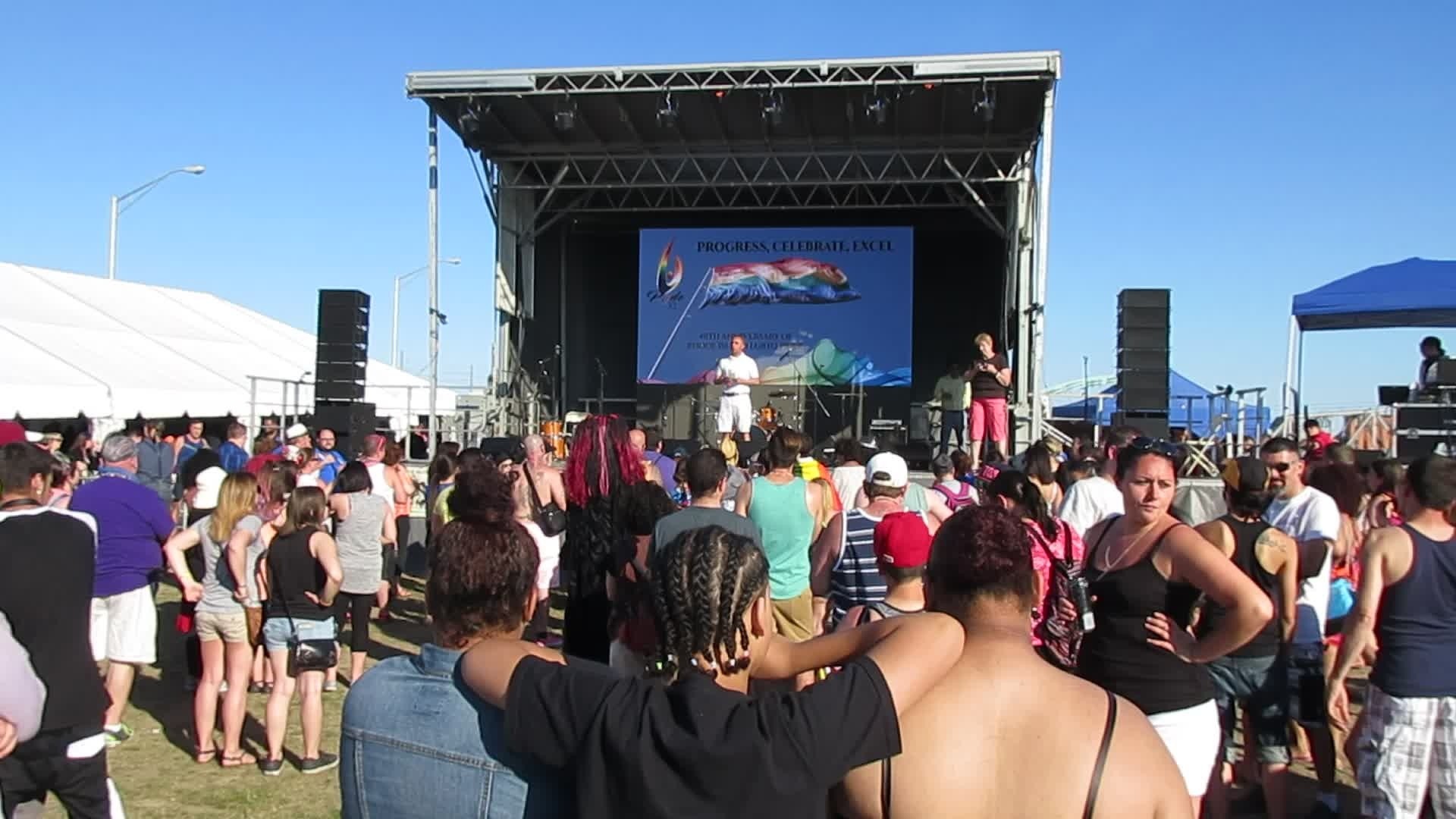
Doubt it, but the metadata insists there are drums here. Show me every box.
[539,418,566,459]
[758,405,780,429]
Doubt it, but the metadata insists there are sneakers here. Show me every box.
[534,631,564,648]
[103,722,135,748]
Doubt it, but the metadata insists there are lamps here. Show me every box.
[553,87,579,135]
[758,80,785,129]
[656,84,683,134]
[969,75,998,121]
[456,97,494,151]
[862,79,893,128]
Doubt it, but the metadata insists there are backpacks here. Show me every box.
[932,481,976,514]
[1022,520,1089,670]
[288,637,337,677]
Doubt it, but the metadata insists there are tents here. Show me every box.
[1280,257,1455,439]
[1051,369,1271,440]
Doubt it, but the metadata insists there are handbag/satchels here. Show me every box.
[523,460,568,536]
[246,606,267,647]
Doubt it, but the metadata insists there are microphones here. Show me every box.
[595,358,607,377]
[779,347,793,363]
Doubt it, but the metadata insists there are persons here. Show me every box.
[0,415,1456,818]
[965,333,1012,472]
[714,333,760,442]
[935,358,972,456]
[1420,336,1447,383]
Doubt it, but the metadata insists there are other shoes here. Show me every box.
[264,747,286,776]
[301,750,340,774]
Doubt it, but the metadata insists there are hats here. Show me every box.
[1221,456,1270,493]
[873,512,935,568]
[865,451,908,488]
[931,453,953,473]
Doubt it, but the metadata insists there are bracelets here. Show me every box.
[994,371,999,376]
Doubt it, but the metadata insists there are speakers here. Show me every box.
[881,442,930,472]
[481,437,526,463]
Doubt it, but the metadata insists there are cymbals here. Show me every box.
[769,388,795,399]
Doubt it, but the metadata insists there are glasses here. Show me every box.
[1129,436,1179,458]
[1268,460,1297,473]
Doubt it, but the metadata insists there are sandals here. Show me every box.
[196,740,222,764]
[220,749,261,768]
